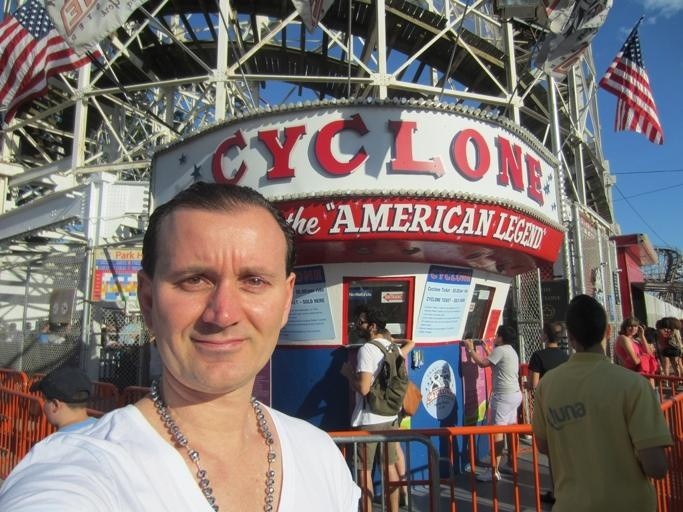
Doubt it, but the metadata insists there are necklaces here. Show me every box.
[149,375,277,512]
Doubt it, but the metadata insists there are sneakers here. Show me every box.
[499,464,513,474]
[541,491,555,504]
[475,468,500,483]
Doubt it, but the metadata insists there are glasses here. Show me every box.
[357,318,373,325]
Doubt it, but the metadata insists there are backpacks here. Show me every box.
[364,340,409,415]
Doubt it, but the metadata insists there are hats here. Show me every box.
[29,365,91,403]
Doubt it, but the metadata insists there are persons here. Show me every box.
[340,302,405,512]
[530,293,672,511]
[28,365,99,433]
[525,318,570,389]
[376,329,416,507]
[461,325,522,483]
[613,315,682,391]
[0,180,363,512]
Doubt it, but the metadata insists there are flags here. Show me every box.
[292,0,337,35]
[597,14,664,146]
[43,0,150,58]
[490,1,613,82]
[0,0,99,124]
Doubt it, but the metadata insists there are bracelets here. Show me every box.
[466,348,475,356]
